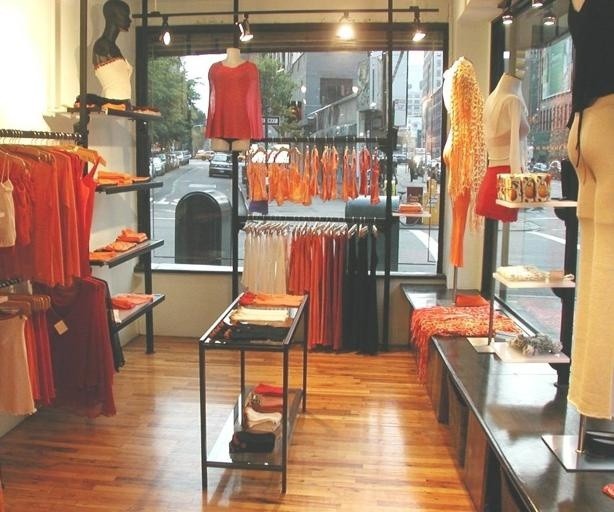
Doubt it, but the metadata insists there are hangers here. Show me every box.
[0,274,52,319]
[242,214,382,241]
[0,125,108,171]
[248,135,382,163]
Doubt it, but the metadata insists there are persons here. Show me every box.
[202,46,263,154]
[89,0,135,100]
[439,57,488,268]
[472,71,531,224]
[562,0,614,423]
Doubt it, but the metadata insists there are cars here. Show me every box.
[196,150,233,177]
[533,159,562,180]
[393,153,441,182]
[149,150,192,179]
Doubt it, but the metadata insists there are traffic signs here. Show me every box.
[261,116,279,126]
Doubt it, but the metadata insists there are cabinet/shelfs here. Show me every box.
[230,129,397,357]
[199,290,309,492]
[399,200,614,512]
[72,93,166,354]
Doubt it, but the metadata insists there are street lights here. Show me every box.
[187,76,203,151]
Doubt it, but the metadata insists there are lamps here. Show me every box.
[149,10,176,46]
[408,14,430,45]
[499,2,518,28]
[231,14,257,45]
[334,11,359,44]
[540,4,556,27]
